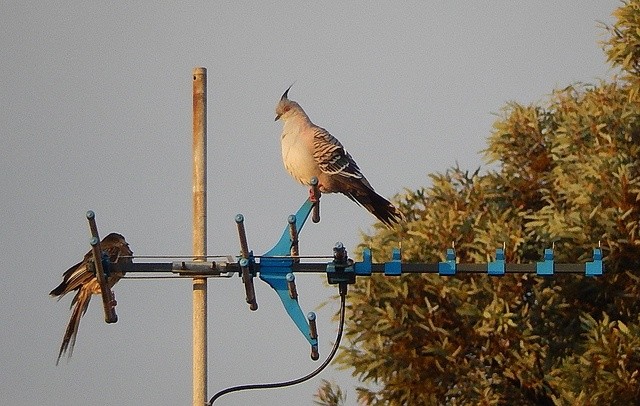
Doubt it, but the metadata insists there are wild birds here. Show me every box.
[49,232,134,368]
[273,81,404,231]
